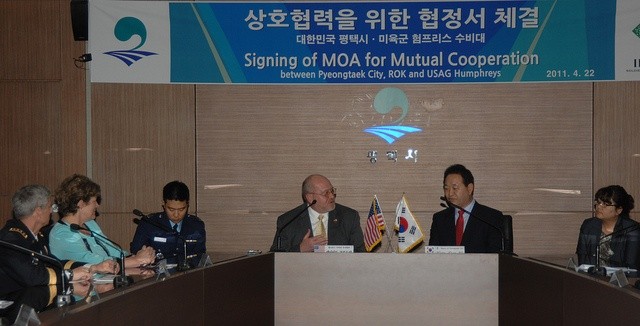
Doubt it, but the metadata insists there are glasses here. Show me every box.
[594,200,615,209]
[307,189,335,197]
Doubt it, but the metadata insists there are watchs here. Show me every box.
[64,268,73,281]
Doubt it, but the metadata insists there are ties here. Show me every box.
[173,223,178,231]
[455,210,463,246]
[316,216,326,237]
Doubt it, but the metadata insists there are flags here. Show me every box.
[393,195,426,254]
[362,196,388,252]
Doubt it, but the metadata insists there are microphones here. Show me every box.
[133,209,192,270]
[439,196,507,255]
[69,224,133,288]
[588,223,640,277]
[267,200,316,252]
[0,235,71,306]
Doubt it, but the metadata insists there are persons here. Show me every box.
[129,180,207,258]
[269,173,366,254]
[427,162,503,253]
[47,174,156,271]
[575,184,640,272]
[0,184,120,291]
